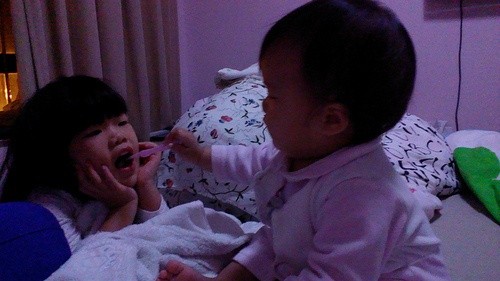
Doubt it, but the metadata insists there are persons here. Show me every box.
[160,0,454,281]
[0,73,170,235]
